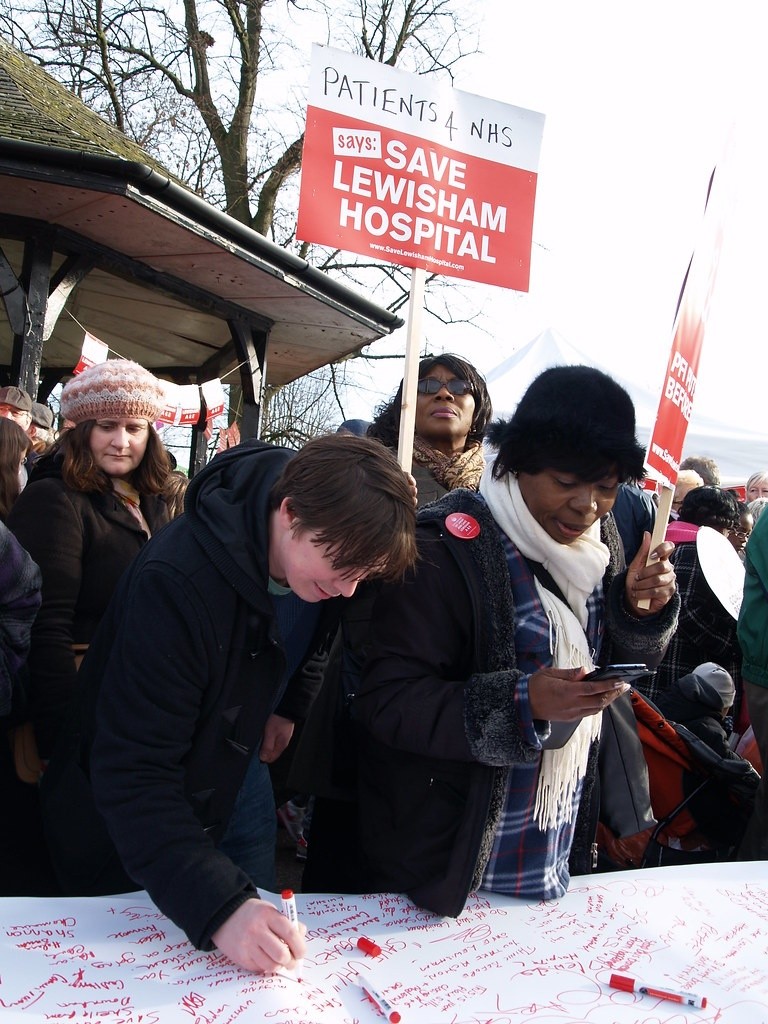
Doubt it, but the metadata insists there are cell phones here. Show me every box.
[582,663,648,681]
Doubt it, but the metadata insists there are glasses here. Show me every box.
[416,377,474,396]
[732,528,751,541]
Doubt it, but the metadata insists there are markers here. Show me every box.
[608,974,708,1009]
[281,890,303,983]
[356,971,401,1024]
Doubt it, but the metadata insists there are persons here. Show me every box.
[38,435,422,974]
[336,419,374,437]
[372,353,493,513]
[288,367,682,919]
[641,457,768,860]
[0,359,192,898]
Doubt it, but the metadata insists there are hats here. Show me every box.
[30,402,53,428]
[0,386,32,412]
[58,359,162,423]
[692,662,735,708]
